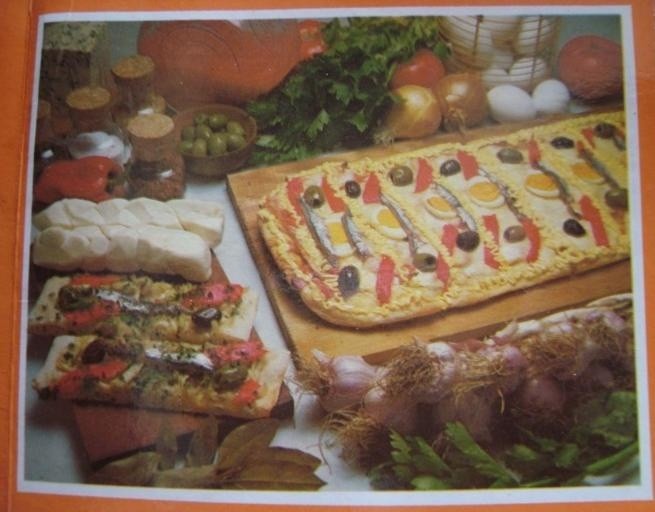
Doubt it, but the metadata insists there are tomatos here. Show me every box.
[390,47,447,90]
[557,36,624,102]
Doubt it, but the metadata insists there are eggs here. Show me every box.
[443,15,571,123]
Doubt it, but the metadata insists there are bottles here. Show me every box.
[37,57,185,201]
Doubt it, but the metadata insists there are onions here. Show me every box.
[372,84,441,146]
[435,71,486,131]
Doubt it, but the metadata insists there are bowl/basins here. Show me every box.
[174,101,254,176]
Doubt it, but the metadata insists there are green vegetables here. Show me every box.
[366,387,640,489]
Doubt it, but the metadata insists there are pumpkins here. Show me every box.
[137,21,301,111]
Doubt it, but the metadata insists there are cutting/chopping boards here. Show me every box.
[226,107,634,371]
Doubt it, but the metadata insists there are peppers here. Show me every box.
[34,156,129,203]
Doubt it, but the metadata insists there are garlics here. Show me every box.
[317,306,634,437]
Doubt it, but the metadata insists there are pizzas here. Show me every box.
[32,334,292,419]
[257,108,630,329]
[29,275,261,345]
